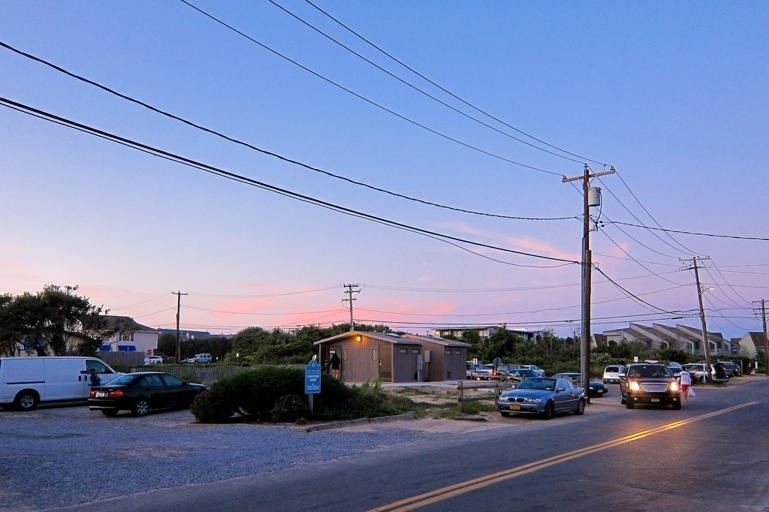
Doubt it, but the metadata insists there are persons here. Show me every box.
[328,353,340,379]
[677,365,696,403]
[89,368,101,390]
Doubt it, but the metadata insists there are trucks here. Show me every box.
[0,356,128,411]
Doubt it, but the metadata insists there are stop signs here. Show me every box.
[147,349,153,355]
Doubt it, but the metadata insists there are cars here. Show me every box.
[180,356,189,364]
[551,371,609,397]
[601,359,742,411]
[464,357,548,382]
[88,371,208,418]
[495,376,590,421]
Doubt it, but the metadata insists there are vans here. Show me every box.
[188,353,213,365]
[144,355,163,365]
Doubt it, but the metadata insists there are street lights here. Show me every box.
[699,285,715,383]
